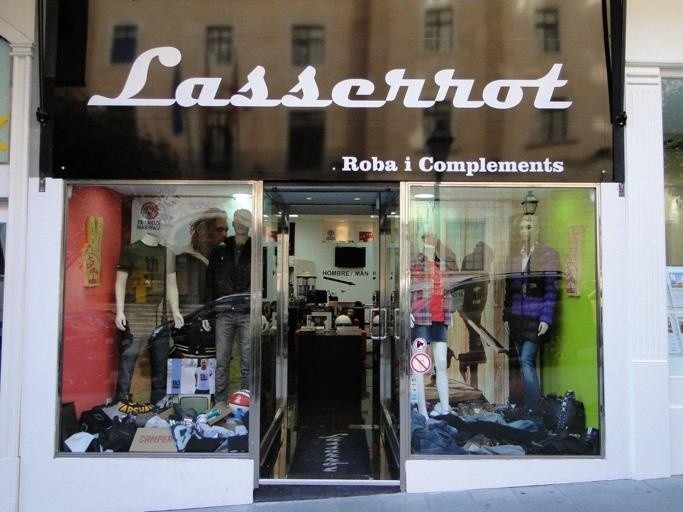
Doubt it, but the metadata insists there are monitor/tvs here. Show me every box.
[335,246,366,267]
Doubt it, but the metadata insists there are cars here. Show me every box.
[138,292,251,389]
[411,271,594,368]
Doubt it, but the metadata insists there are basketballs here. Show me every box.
[228,388,250,417]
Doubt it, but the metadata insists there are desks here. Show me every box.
[297,329,366,402]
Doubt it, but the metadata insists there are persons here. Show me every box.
[176,206,228,304]
[458,240,497,388]
[200,209,269,403]
[114,232,185,405]
[499,214,562,405]
[410,224,453,421]
